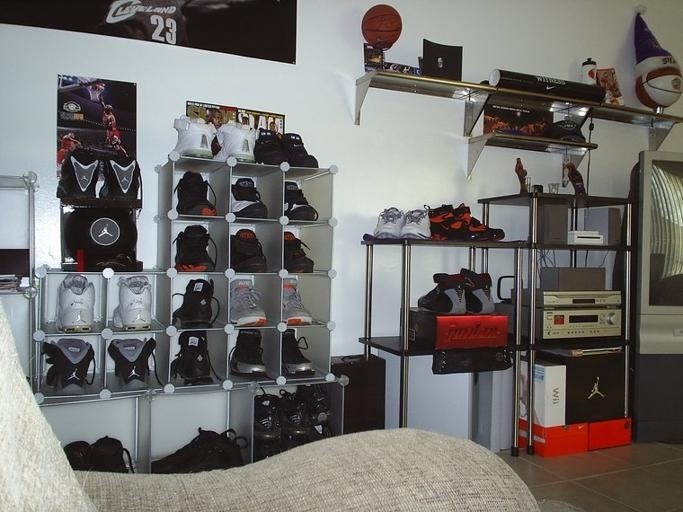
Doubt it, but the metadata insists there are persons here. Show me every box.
[57,130,81,166]
[99,95,128,157]
[270,122,282,139]
[206,108,222,153]
[242,116,249,125]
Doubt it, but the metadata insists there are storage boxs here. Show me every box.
[518,417,588,457]
[534,351,627,425]
[517,356,566,429]
[589,418,633,450]
[399,306,510,353]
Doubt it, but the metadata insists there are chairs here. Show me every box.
[0,303,537,512]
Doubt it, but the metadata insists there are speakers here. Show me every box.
[536,203,569,246]
[539,266,606,292]
[583,207,622,246]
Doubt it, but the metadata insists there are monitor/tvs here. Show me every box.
[612,149,683,355]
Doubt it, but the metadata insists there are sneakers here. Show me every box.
[55,148,145,202]
[172,278,220,328]
[64,436,138,473]
[284,230,314,274]
[418,268,496,316]
[170,330,222,386]
[214,122,257,163]
[231,177,268,219]
[374,206,431,241]
[111,275,152,331]
[254,128,319,168]
[174,170,217,216]
[230,278,267,327]
[149,426,250,476]
[107,337,166,391]
[230,229,267,273]
[284,181,319,221]
[55,273,96,334]
[427,203,506,242]
[283,275,313,326]
[228,327,276,381]
[39,338,97,396]
[172,224,219,272]
[167,115,217,159]
[256,384,336,460]
[282,328,317,378]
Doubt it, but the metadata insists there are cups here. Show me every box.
[532,184,542,192]
[547,183,560,195]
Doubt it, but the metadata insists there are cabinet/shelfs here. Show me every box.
[477,191,635,457]
[35,154,335,399]
[353,70,682,188]
[356,235,523,455]
[0,174,348,476]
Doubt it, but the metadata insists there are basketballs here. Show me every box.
[362,5,402,50]
[635,67,682,108]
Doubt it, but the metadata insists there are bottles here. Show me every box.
[581,57,597,85]
[526,177,531,193]
